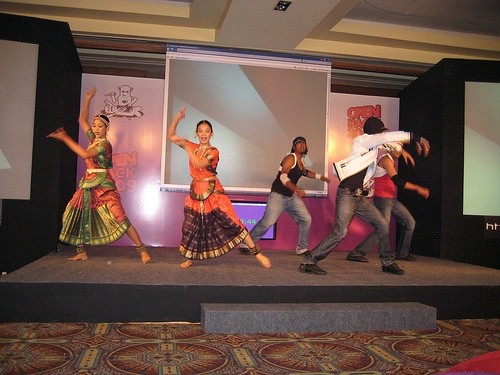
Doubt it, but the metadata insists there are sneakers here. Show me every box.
[298,263,327,275]
[383,264,404,275]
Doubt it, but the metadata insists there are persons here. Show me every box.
[299,116,430,275]
[167,107,272,268]
[47,87,151,264]
[238,136,331,258]
[346,143,430,262]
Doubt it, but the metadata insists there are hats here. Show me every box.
[363,117,388,134]
[291,137,306,152]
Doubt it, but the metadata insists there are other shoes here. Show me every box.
[397,255,416,261]
[300,250,312,258]
[346,254,369,262]
[239,247,250,255]
